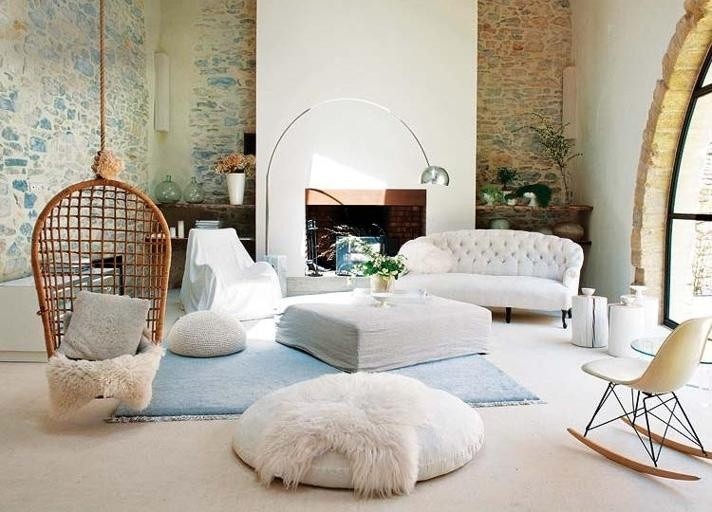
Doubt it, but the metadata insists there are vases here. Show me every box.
[225,172,245,205]
[368,275,392,293]
[553,166,577,208]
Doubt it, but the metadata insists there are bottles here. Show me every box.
[568,284,660,358]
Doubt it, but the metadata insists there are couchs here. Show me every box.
[386,225,586,328]
[166,307,249,358]
[226,368,489,500]
[179,226,284,323]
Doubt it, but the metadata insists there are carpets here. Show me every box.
[98,311,546,412]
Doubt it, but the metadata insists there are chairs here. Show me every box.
[557,310,711,485]
[31,1,176,413]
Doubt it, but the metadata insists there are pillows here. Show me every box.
[59,287,152,363]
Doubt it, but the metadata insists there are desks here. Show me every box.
[628,330,711,371]
[604,303,641,359]
[274,289,493,374]
[569,297,610,348]
[285,276,378,295]
[621,293,660,332]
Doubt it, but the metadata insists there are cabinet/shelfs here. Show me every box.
[142,201,255,247]
[476,204,594,247]
[0,266,121,362]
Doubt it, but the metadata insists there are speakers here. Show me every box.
[562,66,577,140]
[154,53,171,133]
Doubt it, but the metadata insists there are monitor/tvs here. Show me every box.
[243,132,256,154]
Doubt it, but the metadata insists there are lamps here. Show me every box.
[264,93,450,282]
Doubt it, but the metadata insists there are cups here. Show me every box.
[490,217,509,229]
[178,220,184,238]
[170,227,176,237]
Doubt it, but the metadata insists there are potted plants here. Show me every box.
[495,166,518,196]
[503,193,518,206]
[518,182,552,207]
[478,182,504,206]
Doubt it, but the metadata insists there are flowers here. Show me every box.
[513,107,584,198]
[215,151,254,180]
[310,219,411,281]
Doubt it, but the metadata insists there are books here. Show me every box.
[194,220,222,229]
[49,258,90,275]
